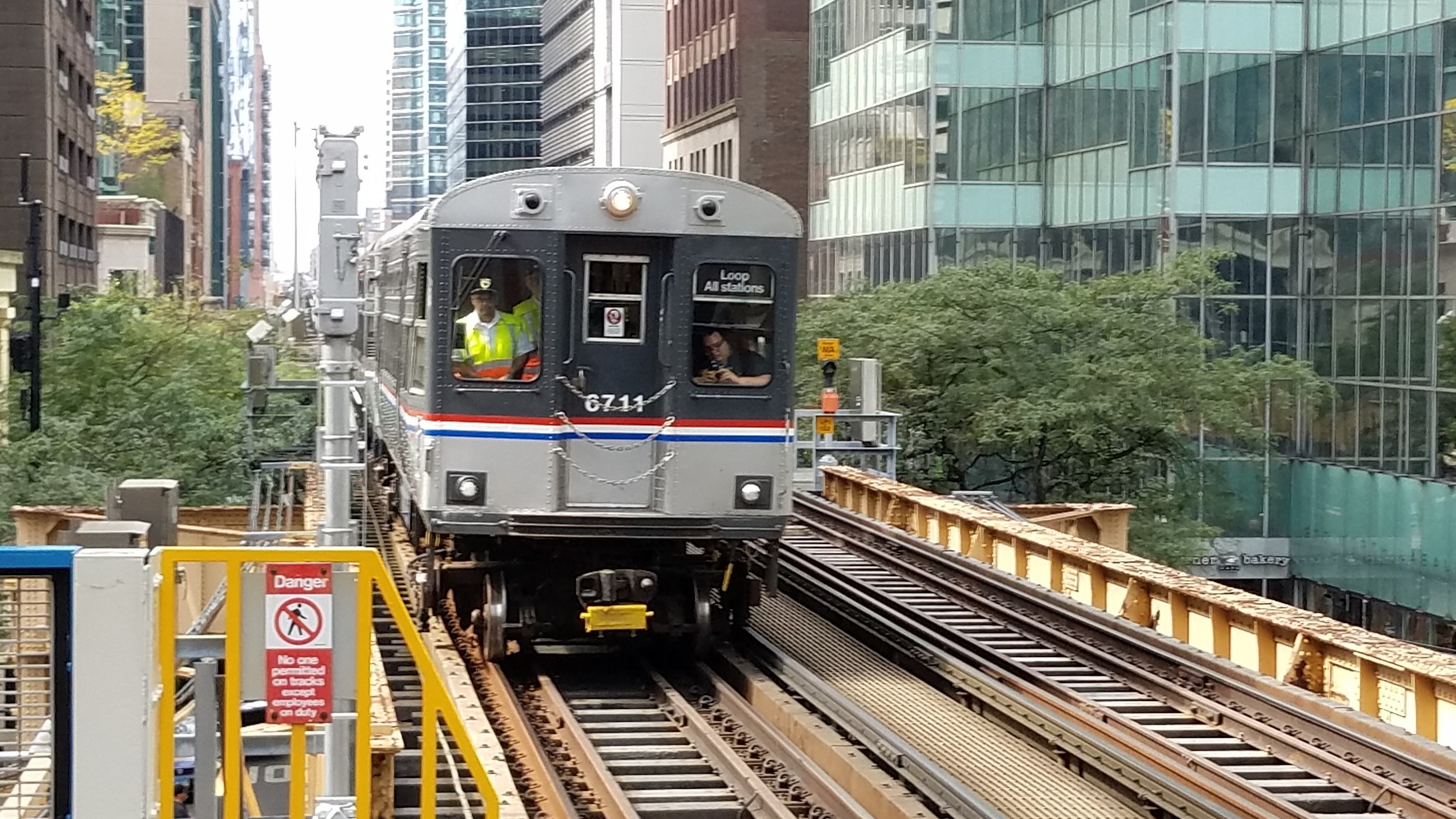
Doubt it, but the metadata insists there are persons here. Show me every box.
[692,327,773,384]
[450,261,543,380]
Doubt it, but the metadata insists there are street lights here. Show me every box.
[7,293,70,481]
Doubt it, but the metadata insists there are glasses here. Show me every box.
[704,338,725,356]
[471,297,494,303]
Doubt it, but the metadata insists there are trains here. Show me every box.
[352,166,804,664]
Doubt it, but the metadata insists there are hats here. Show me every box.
[470,277,498,294]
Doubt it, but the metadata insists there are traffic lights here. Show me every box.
[822,361,836,386]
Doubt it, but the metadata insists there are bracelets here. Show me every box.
[508,372,516,379]
